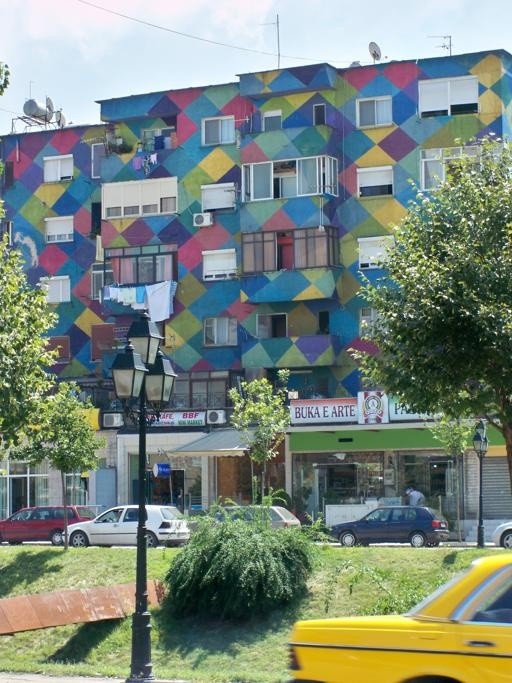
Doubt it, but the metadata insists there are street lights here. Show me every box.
[472,418,489,549]
[110,307,182,682]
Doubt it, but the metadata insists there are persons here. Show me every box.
[404,486,425,506]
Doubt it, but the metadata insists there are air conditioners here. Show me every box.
[192,210,213,228]
[205,409,227,424]
[102,413,125,428]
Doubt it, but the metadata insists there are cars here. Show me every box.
[62,503,189,546]
[0,506,96,546]
[284,551,512,683]
[326,505,451,548]
[189,505,301,532]
[492,521,512,548]
